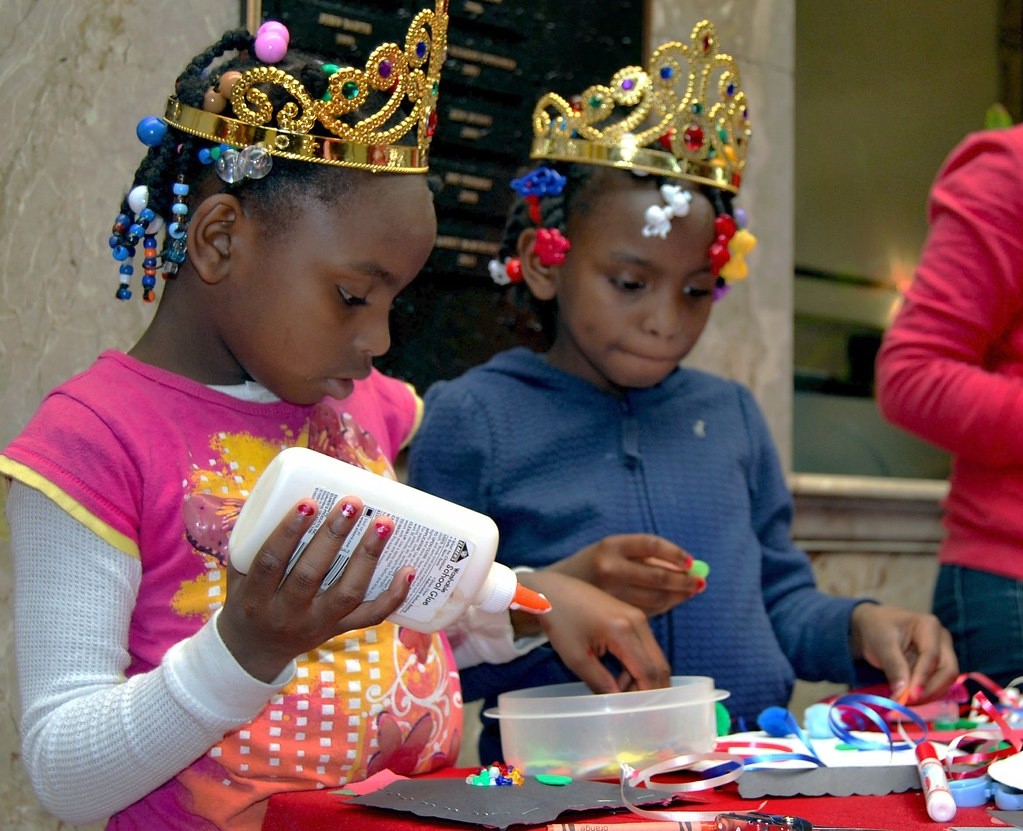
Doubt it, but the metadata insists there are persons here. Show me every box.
[0,30,673,831]
[877,124,1023,751]
[406,108,959,769]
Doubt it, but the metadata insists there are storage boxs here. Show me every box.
[484,675,731,775]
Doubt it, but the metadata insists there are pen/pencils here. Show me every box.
[546,818,751,831]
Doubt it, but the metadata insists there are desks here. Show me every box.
[258,766,1023,831]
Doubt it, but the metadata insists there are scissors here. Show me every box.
[947,770,1022,812]
